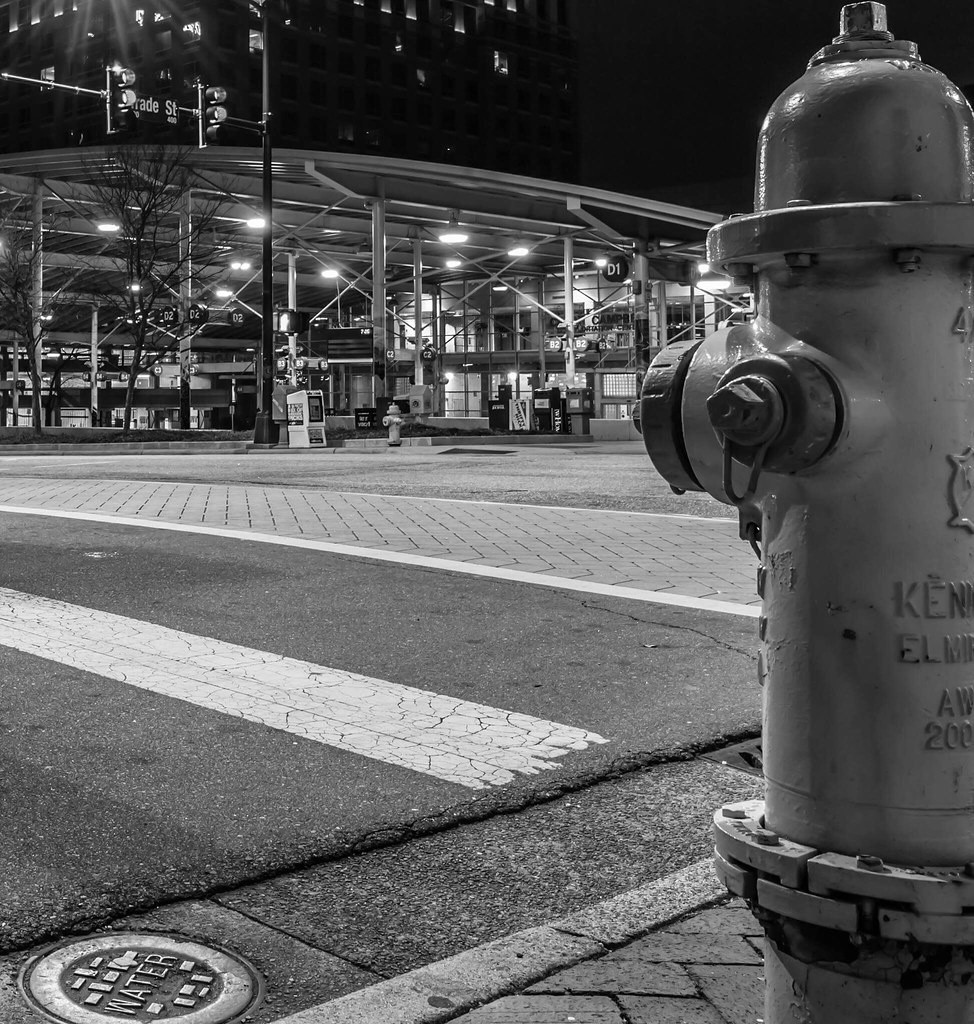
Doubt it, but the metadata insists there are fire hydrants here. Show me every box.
[383,401,407,447]
[633,1,974,1024]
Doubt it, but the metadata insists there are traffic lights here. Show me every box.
[274,306,300,336]
[110,65,138,134]
[200,86,229,146]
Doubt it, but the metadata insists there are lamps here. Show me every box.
[698,250,709,273]
[241,245,250,271]
[247,200,266,228]
[493,275,508,292]
[231,245,240,270]
[696,269,731,290]
[126,276,143,291]
[97,207,120,233]
[508,234,528,256]
[440,212,468,243]
[40,309,54,321]
